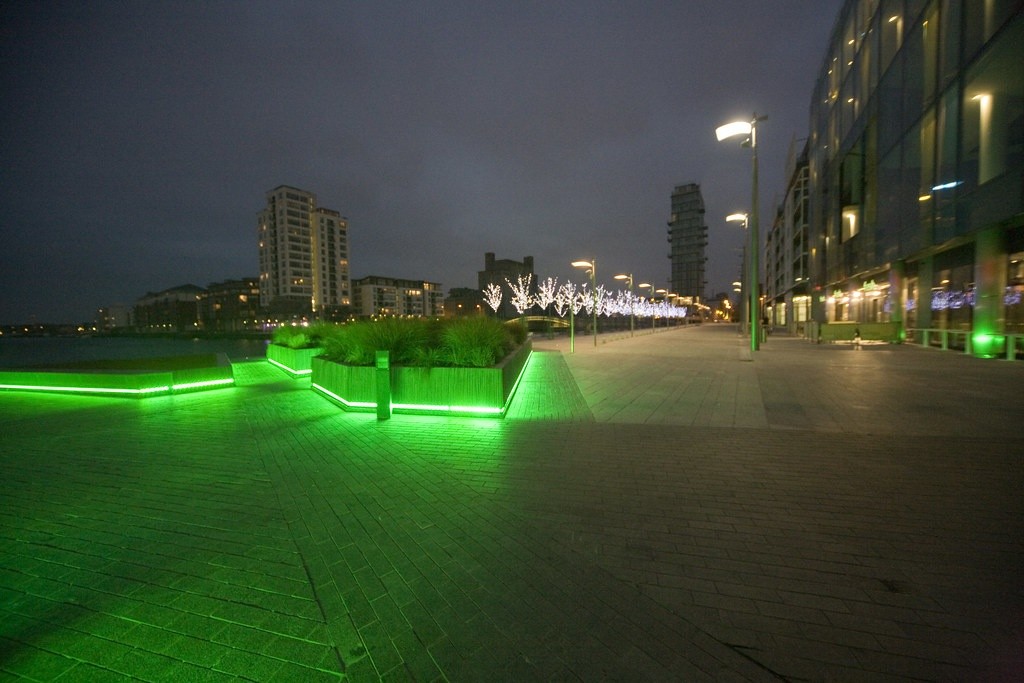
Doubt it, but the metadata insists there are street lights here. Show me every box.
[571,260,597,346]
[725,212,751,330]
[668,292,680,329]
[656,289,669,331]
[613,272,634,338]
[714,121,762,354]
[638,283,654,333]
